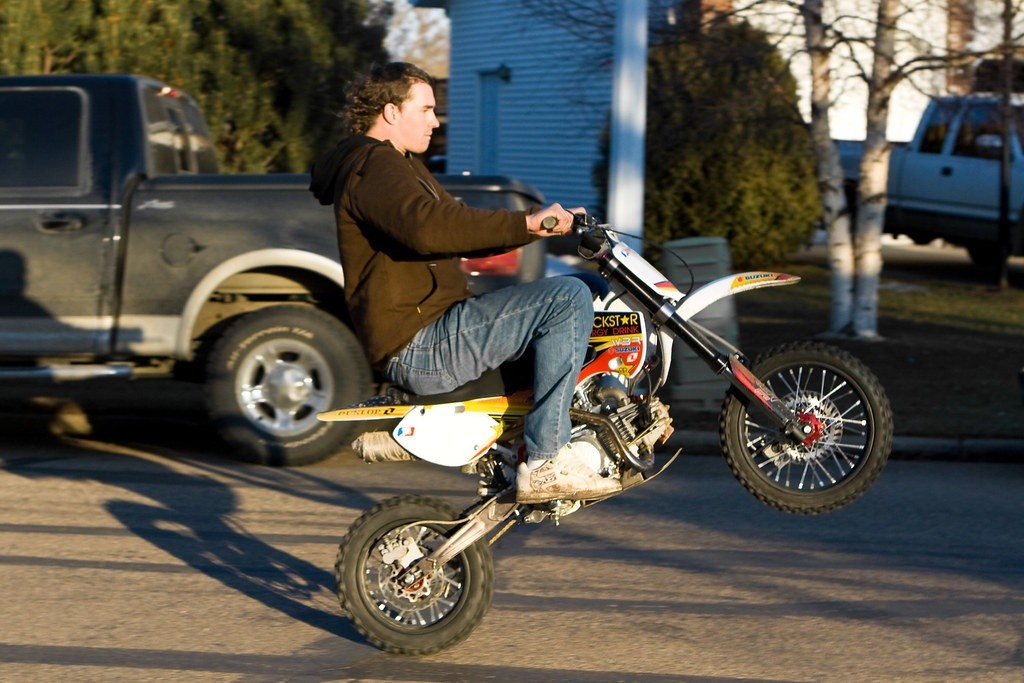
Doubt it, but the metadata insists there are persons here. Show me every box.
[307,62,621,503]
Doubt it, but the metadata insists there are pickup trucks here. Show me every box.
[830,96,1024,263]
[0,74,546,466]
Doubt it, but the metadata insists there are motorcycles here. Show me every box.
[315,211,894,657]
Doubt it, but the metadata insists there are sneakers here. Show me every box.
[516,457,623,503]
